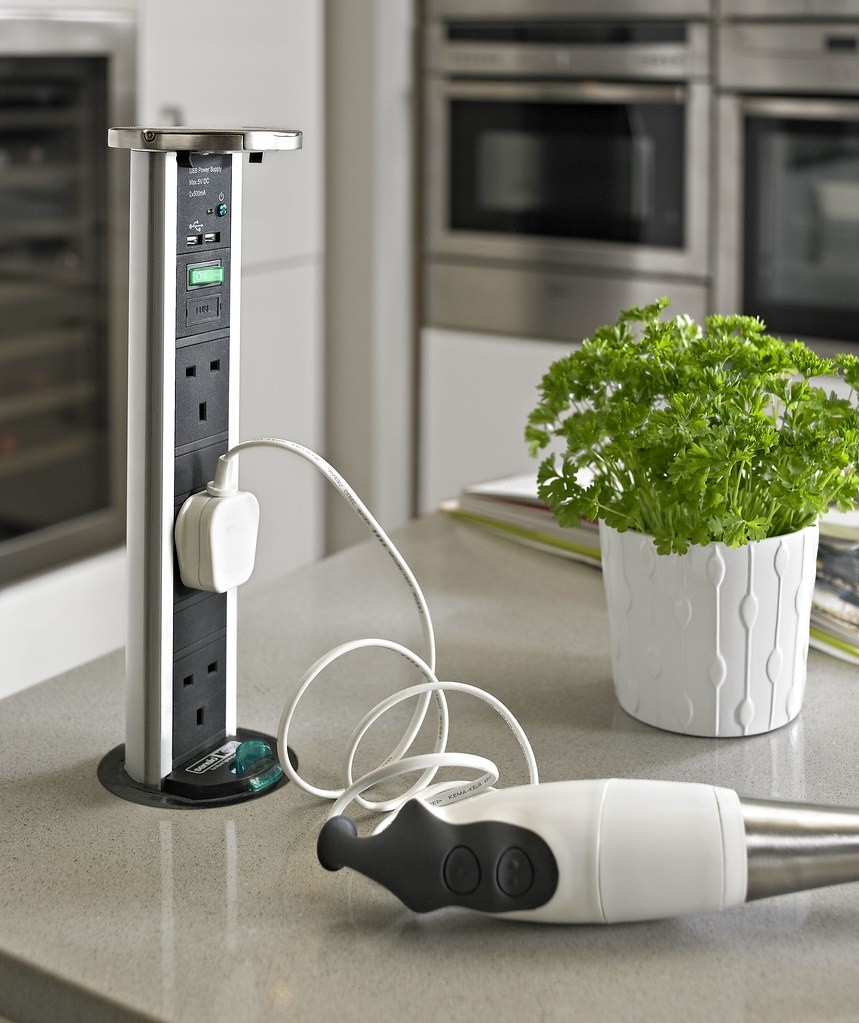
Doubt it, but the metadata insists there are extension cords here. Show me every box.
[123,145,245,794]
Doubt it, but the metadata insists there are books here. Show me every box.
[437,465,859,665]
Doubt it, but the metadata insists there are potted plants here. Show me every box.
[526,295,859,737]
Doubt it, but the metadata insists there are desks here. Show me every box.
[1,512,859,1023]
